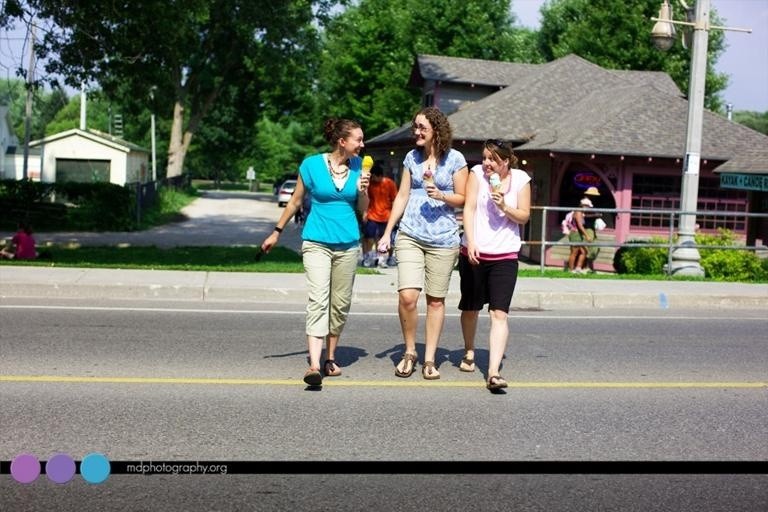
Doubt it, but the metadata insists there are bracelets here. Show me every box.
[274,226,283,233]
[442,193,446,201]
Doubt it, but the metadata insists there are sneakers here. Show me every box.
[362,254,396,268]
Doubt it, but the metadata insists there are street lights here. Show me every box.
[650,1,713,276]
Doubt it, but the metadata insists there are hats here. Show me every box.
[580,198,594,208]
[584,187,600,196]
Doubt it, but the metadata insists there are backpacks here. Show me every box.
[561,210,576,235]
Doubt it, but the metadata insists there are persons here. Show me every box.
[261,118,375,384]
[458,138,533,390]
[364,165,396,268]
[365,228,396,266]
[376,109,467,378]
[562,199,590,274]
[580,187,606,272]
[1,223,38,260]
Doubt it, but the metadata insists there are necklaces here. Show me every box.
[328,156,347,179]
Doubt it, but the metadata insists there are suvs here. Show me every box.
[273,173,298,195]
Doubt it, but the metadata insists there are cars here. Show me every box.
[278,179,297,206]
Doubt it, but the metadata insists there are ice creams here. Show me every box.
[361,154,374,191]
[423,169,433,190]
[490,172,500,201]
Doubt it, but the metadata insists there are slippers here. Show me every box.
[460,356,475,372]
[395,353,418,377]
[325,359,341,375]
[303,366,323,385]
[487,375,507,390]
[422,361,439,380]
